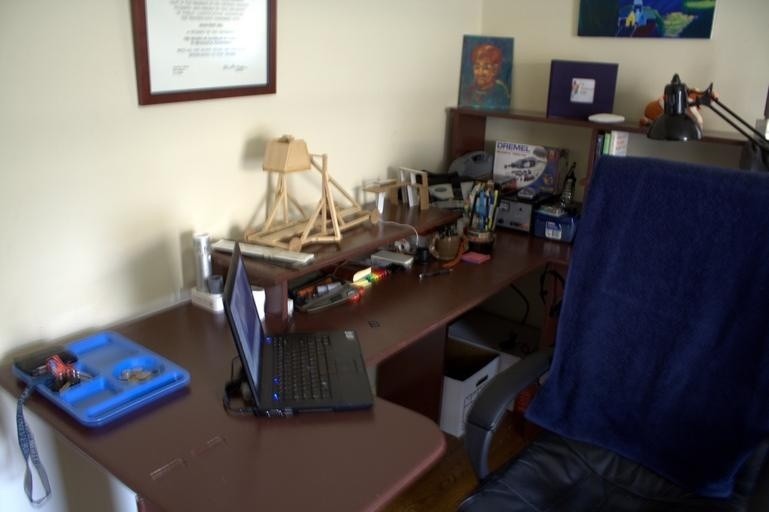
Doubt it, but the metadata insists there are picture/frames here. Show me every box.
[129,0,277,106]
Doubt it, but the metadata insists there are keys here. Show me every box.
[58,369,82,394]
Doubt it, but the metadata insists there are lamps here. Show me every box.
[645,73,769,172]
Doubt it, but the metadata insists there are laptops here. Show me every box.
[222,242,374,418]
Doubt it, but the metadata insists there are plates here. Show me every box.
[588,113,626,125]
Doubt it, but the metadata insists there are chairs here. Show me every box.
[453,154,769,512]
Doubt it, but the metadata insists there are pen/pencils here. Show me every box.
[418,268,453,279]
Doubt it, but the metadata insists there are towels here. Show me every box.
[523,152,769,498]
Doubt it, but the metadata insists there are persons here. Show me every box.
[457,43,511,111]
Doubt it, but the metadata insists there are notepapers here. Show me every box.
[459,252,490,265]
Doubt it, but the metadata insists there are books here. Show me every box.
[608,130,629,157]
[321,257,372,282]
[602,132,611,156]
[592,133,603,167]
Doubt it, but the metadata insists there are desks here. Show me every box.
[0,195,575,512]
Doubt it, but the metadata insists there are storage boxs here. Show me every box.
[447,304,543,414]
[438,335,501,439]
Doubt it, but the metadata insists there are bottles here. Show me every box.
[560,161,577,205]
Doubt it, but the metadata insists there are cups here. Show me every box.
[208,275,224,294]
[430,232,462,261]
[252,286,266,320]
[417,248,429,265]
